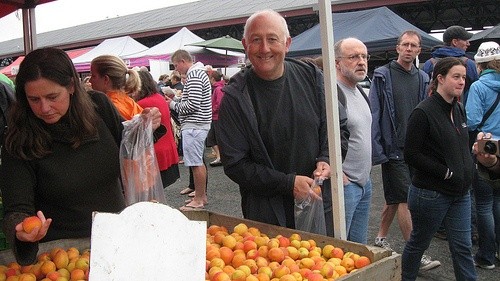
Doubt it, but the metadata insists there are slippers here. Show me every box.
[179,198,209,211]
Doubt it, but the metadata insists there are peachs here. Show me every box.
[0,245,90,281]
[312,188,321,196]
[205,223,370,281]
[22,216,42,234]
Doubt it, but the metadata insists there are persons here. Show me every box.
[400,57,477,281]
[423,26,478,107]
[368,31,440,271]
[0,46,231,266]
[334,37,374,244]
[216,10,350,237]
[465,42,500,270]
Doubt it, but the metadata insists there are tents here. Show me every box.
[286,6,500,61]
[1,27,247,77]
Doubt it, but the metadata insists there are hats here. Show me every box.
[474,41,500,63]
[443,26,473,42]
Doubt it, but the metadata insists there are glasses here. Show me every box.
[338,54,370,63]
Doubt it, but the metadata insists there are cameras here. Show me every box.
[478,139,500,156]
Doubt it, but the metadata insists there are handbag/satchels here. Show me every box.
[119,111,165,206]
[293,171,326,237]
[470,128,500,193]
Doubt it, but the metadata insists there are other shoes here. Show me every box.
[209,157,222,166]
[181,186,195,197]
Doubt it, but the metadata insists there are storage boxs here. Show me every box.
[0,199,402,281]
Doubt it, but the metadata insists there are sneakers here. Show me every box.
[472,248,500,269]
[372,236,398,256]
[419,254,441,272]
